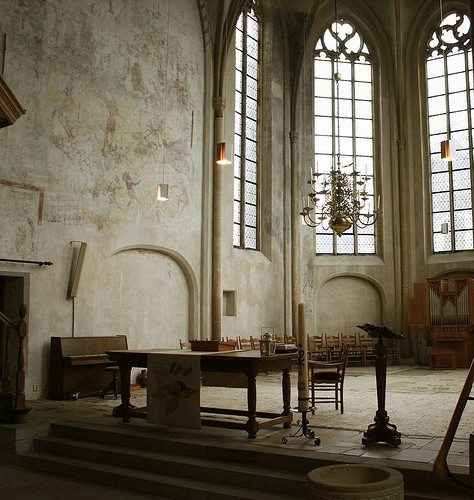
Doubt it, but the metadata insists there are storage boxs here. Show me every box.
[276,344,298,353]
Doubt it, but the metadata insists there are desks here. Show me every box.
[104,348,292,439]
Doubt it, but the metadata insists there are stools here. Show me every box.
[428,349,456,370]
[100,366,125,399]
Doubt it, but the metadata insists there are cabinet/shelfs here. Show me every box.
[424,276,474,368]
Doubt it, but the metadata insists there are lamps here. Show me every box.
[157,0,171,202]
[440,0,455,160]
[216,0,232,165]
[299,0,384,238]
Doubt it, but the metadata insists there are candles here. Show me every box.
[302,154,381,211]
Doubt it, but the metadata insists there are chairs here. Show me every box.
[307,343,350,415]
[179,333,399,369]
